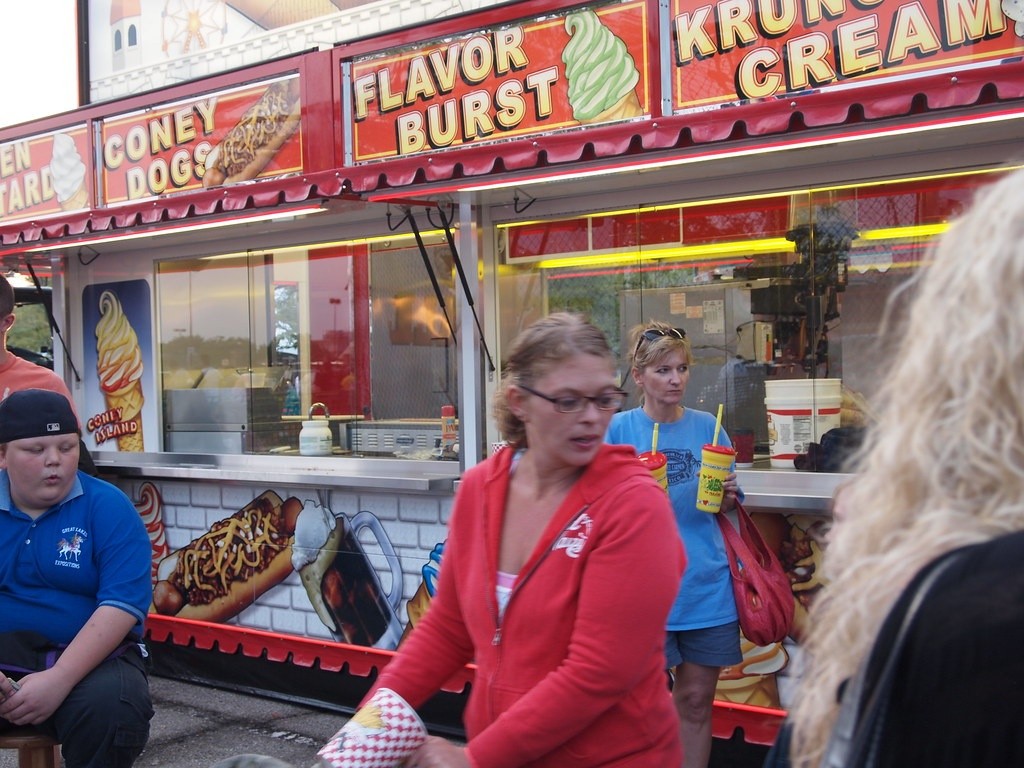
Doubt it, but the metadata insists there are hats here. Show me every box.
[0,389,79,444]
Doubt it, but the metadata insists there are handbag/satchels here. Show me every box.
[715,491,795,647]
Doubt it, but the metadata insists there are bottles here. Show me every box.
[441,405,456,460]
[299,419,333,456]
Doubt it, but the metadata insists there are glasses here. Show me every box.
[517,385,628,413]
[634,328,686,361]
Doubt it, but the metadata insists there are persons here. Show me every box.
[600,320,746,768]
[0,388,156,768]
[357,316,685,768]
[769,158,1024,768]
[0,274,82,430]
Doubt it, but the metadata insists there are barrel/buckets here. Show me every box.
[763,379,842,469]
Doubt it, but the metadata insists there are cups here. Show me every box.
[731,426,755,468]
[696,444,736,513]
[636,451,669,496]
[315,688,428,768]
